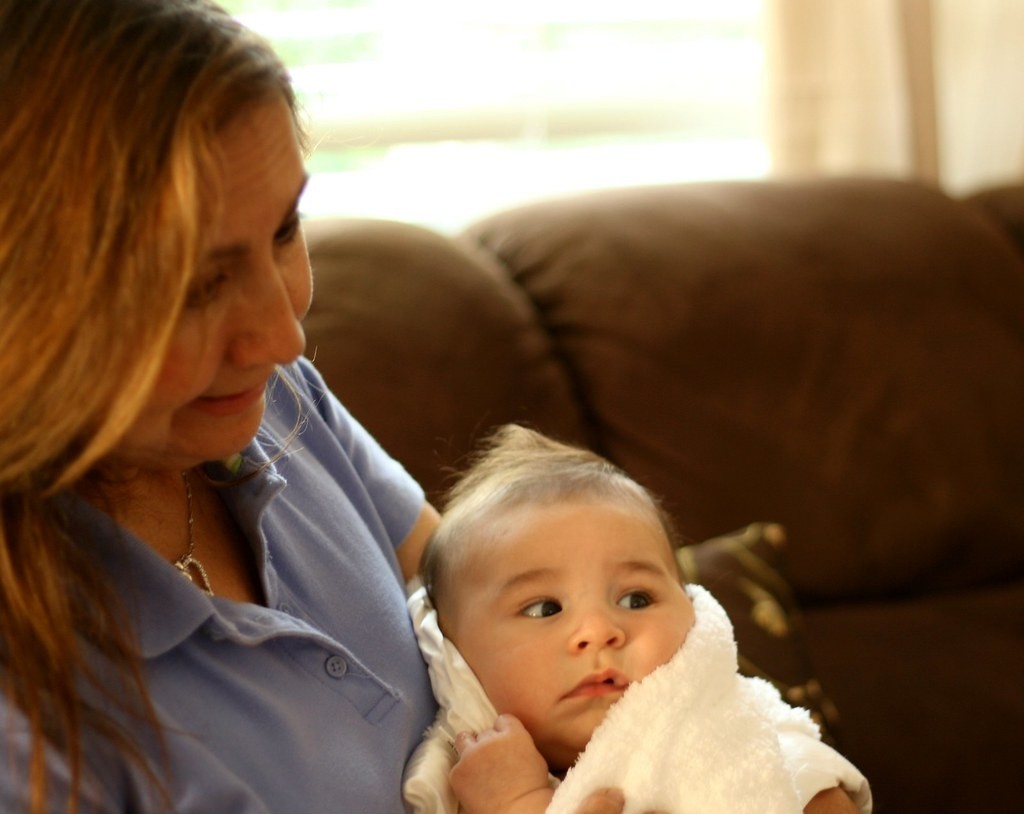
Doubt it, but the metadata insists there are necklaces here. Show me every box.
[169,470,217,598]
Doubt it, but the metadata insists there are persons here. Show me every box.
[1,0,867,814]
[401,422,872,814]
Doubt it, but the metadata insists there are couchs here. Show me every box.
[280,172,1024,814]
